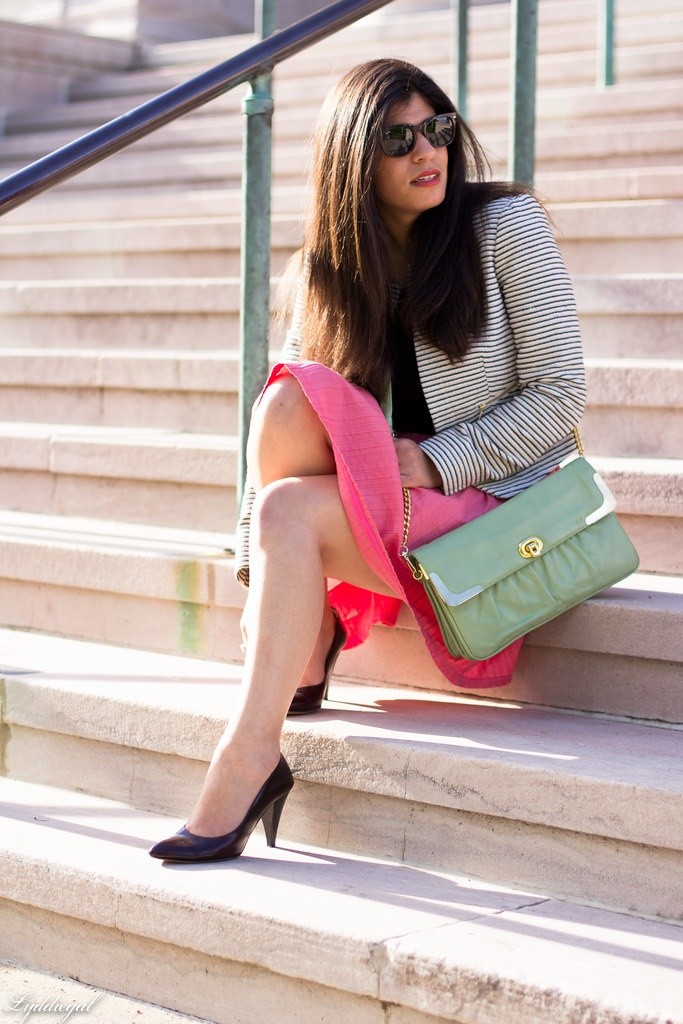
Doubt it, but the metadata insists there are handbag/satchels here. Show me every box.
[406,457,641,661]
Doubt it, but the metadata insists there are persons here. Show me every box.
[148,58,586,863]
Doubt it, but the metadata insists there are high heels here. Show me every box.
[146,754,294,863]
[285,606,346,715]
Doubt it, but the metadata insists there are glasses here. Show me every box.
[378,113,457,157]
